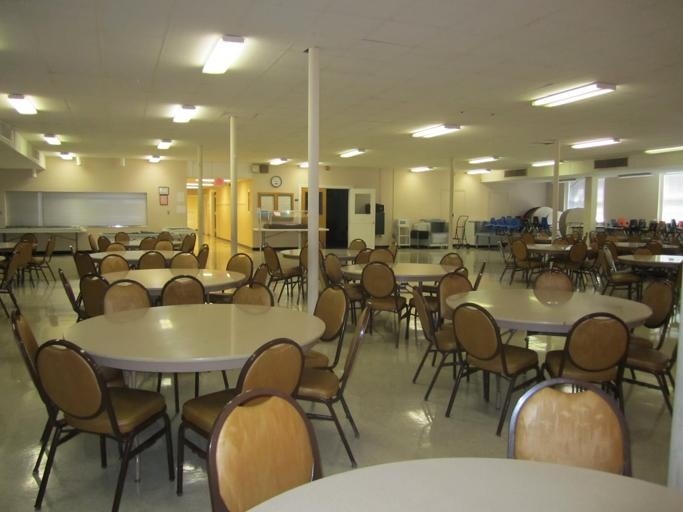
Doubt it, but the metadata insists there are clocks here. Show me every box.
[270,175,284,188]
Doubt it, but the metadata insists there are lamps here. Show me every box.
[149,153,162,164]
[269,157,289,167]
[531,158,565,168]
[527,80,620,109]
[172,102,200,124]
[42,131,63,146]
[296,159,324,168]
[58,150,76,161]
[571,135,627,150]
[156,137,175,151]
[409,165,435,174]
[6,91,40,116]
[467,155,501,166]
[411,122,461,140]
[199,31,247,77]
[339,146,368,158]
[465,167,493,176]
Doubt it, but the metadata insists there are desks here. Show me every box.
[61,303,328,496]
[91,268,247,297]
[340,262,460,295]
[0,239,20,250]
[525,243,577,255]
[244,454,665,512]
[444,287,655,410]
[533,235,563,243]
[616,254,683,270]
[280,248,363,262]
[87,249,183,263]
[109,239,185,248]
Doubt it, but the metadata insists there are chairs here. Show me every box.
[206,252,255,298]
[176,335,306,498]
[139,236,158,250]
[168,251,201,269]
[105,241,126,251]
[415,252,464,296]
[87,233,97,251]
[73,249,98,305]
[160,273,209,307]
[628,278,677,352]
[58,267,89,322]
[32,338,177,512]
[153,239,175,251]
[114,231,130,242]
[525,268,575,348]
[136,250,168,270]
[196,243,209,269]
[294,303,375,468]
[645,236,667,256]
[348,238,367,250]
[508,377,636,478]
[506,225,683,243]
[368,247,395,263]
[435,272,475,330]
[607,239,619,262]
[616,342,682,417]
[553,242,588,293]
[229,281,275,308]
[324,252,363,327]
[156,231,173,241]
[354,247,374,265]
[11,306,130,477]
[443,302,541,438]
[631,245,655,257]
[98,253,130,275]
[507,237,544,291]
[79,272,111,319]
[0,249,24,320]
[672,260,682,309]
[600,243,645,302]
[262,245,302,303]
[411,285,471,402]
[304,239,325,249]
[539,310,632,415]
[303,281,352,372]
[182,232,197,254]
[97,235,111,253]
[32,233,58,285]
[12,230,37,289]
[613,242,679,251]
[296,245,323,305]
[408,266,469,338]
[473,261,487,291]
[497,240,514,281]
[360,260,409,349]
[387,240,398,258]
[252,263,275,288]
[99,279,156,315]
[206,386,326,512]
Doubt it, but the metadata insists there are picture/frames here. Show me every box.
[158,194,168,206]
[257,191,276,221]
[276,192,294,222]
[158,186,171,195]
